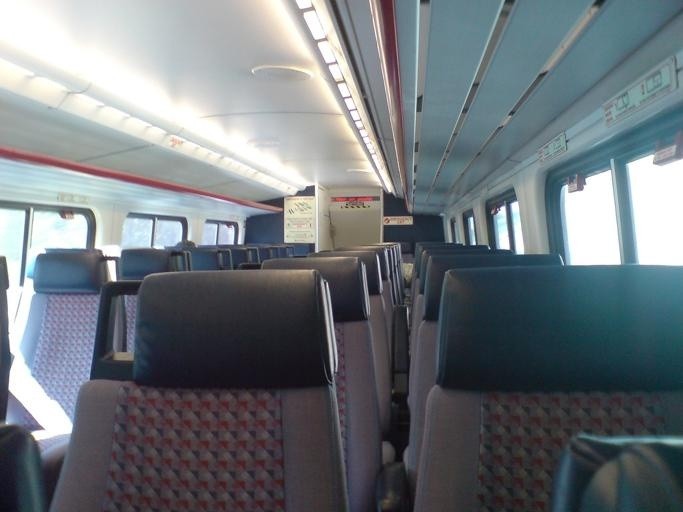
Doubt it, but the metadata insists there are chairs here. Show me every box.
[0,243,404,512]
[404,241,680,512]
